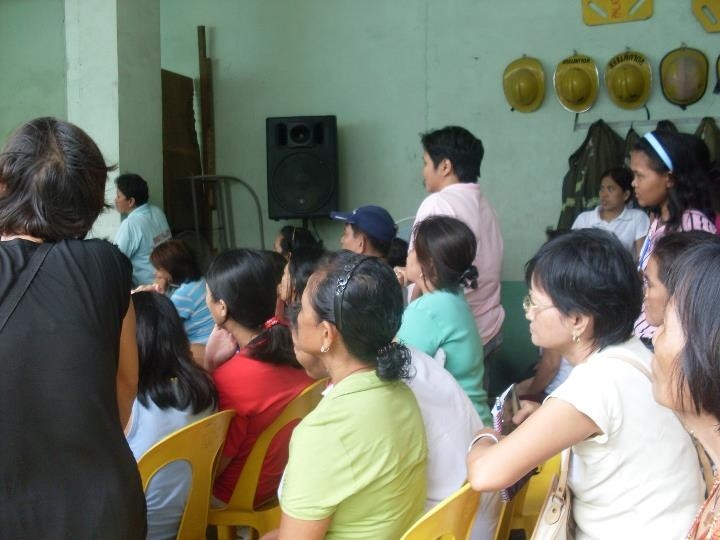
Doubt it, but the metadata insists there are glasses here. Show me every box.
[522,295,556,314]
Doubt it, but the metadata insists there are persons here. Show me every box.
[0,115,152,540]
[89,123,720,540]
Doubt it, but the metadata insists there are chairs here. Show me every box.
[123,373,566,540]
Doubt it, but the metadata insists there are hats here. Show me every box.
[330,206,396,243]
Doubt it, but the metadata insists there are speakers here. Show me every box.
[266,115,338,222]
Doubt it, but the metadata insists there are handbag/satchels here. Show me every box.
[528,474,577,540]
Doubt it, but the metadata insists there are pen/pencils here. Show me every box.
[495,396,501,411]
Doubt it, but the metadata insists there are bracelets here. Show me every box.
[468,433,500,452]
